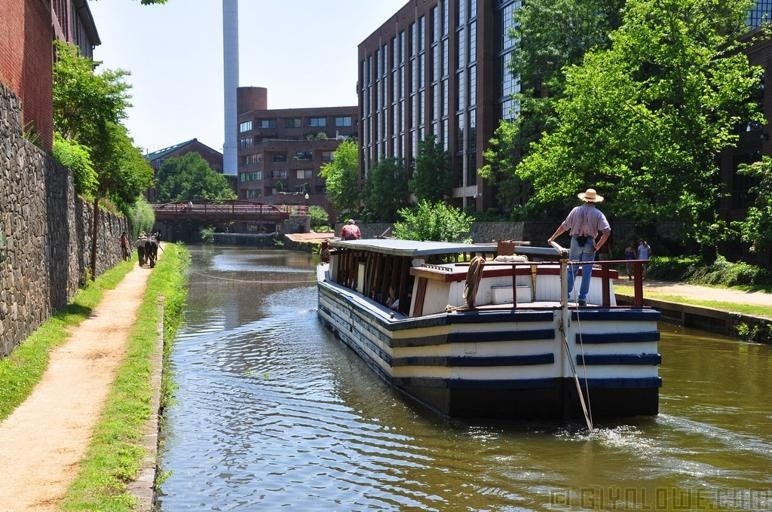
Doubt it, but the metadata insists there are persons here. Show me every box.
[634,239,652,281]
[382,282,401,313]
[187,200,194,211]
[120,231,131,263]
[339,218,362,241]
[546,187,612,307]
[135,234,148,267]
[624,241,638,281]
[318,241,331,266]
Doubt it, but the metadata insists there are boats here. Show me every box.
[316,236,662,424]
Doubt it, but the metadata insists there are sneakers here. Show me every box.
[579,300,586,306]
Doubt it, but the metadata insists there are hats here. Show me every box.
[577,189,604,203]
